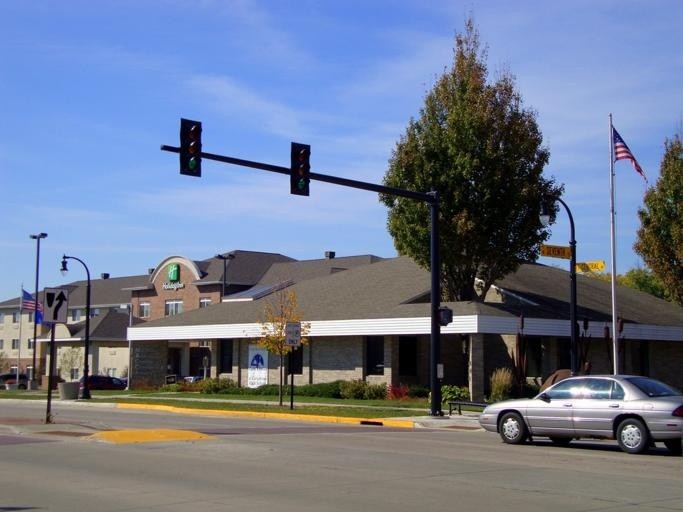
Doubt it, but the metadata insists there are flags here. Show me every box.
[611,124,648,184]
[20,290,44,311]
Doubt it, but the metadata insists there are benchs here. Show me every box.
[448,401,488,415]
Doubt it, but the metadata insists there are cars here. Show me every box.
[0,374,28,390]
[478,374,683,454]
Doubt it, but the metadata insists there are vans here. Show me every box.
[77,375,127,390]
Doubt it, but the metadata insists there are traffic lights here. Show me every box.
[179,117,202,177]
[290,141,311,196]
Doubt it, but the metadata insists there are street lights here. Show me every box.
[59,253,91,399]
[539,192,579,377]
[27,232,48,391]
[215,253,235,297]
